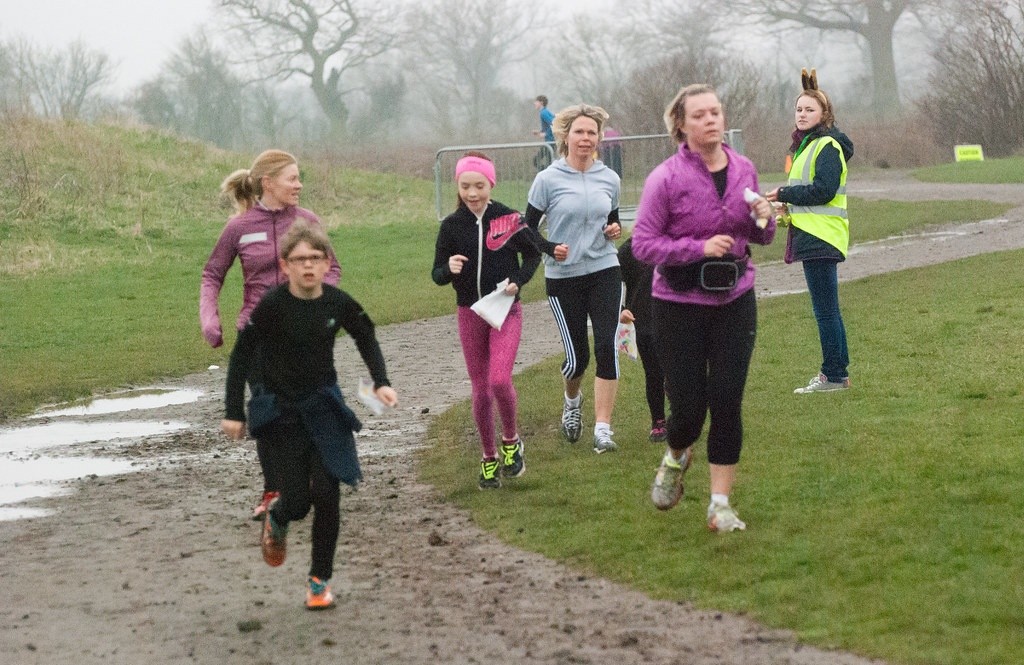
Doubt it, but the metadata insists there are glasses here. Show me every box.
[283,255,328,265]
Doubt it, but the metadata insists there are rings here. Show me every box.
[614,233,616,236]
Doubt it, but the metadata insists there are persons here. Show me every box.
[526,105,622,454]
[631,85,776,533]
[532,95,558,171]
[201,151,342,518]
[595,121,621,178]
[766,67,854,394]
[432,152,542,490]
[223,223,397,609]
[618,238,667,442]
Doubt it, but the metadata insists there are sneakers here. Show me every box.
[305,577,335,609]
[562,392,584,443]
[842,377,850,389]
[593,428,618,454]
[478,457,502,491]
[252,491,280,521]
[650,419,667,443]
[707,502,746,532]
[793,373,843,394]
[500,438,525,479]
[262,496,287,566]
[653,446,692,510]
